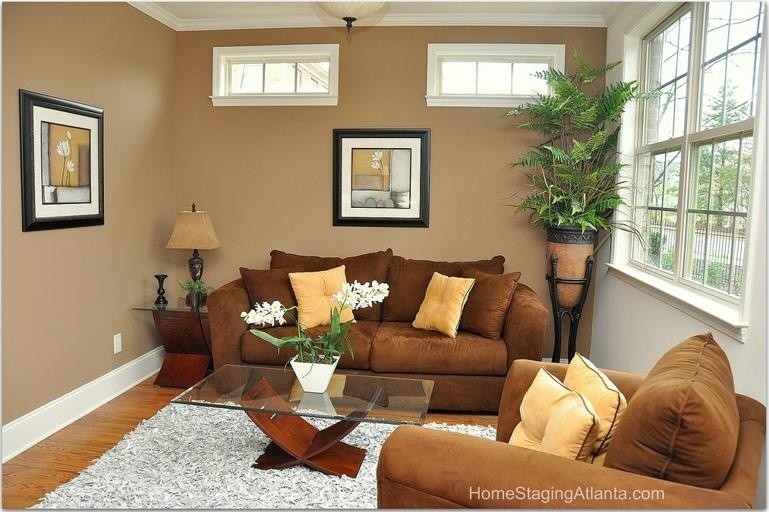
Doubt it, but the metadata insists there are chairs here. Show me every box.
[373,357,763,510]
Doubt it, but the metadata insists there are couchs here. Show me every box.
[206,274,549,415]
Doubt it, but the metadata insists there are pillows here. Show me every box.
[505,368,597,462]
[412,271,475,340]
[239,264,320,326]
[287,264,358,330]
[458,267,523,341]
[269,247,393,320]
[602,331,740,488]
[378,255,505,322]
[559,349,630,465]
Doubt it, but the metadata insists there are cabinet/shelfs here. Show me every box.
[132,295,211,391]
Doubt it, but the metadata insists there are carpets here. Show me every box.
[30,400,498,508]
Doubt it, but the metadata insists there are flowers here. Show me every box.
[231,280,390,364]
[369,150,387,190]
[56,130,76,186]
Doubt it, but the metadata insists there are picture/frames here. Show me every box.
[333,126,431,229]
[17,88,107,232]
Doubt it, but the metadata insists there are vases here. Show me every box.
[298,393,336,414]
[287,355,340,396]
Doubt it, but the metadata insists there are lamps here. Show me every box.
[167,201,220,305]
[315,2,389,33]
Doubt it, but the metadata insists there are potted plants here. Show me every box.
[497,41,669,308]
